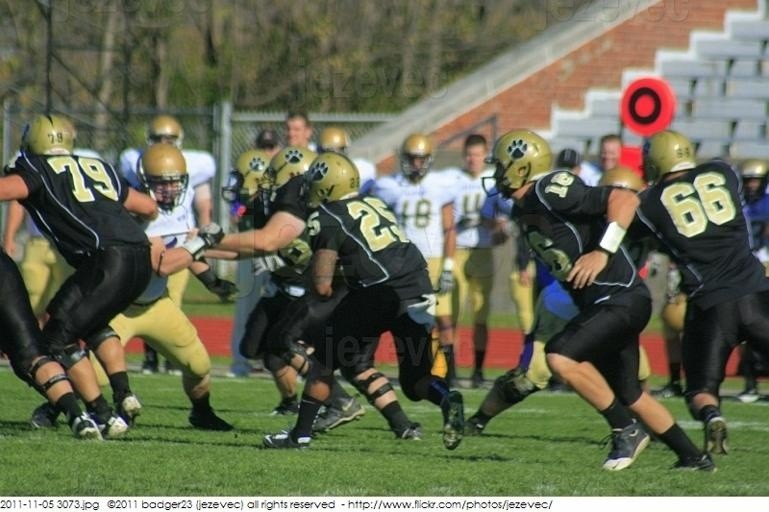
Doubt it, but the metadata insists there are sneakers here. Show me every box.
[444,374,460,389]
[96,409,129,439]
[269,404,301,418]
[440,390,466,451]
[120,394,142,425]
[139,359,159,374]
[71,413,104,441]
[311,393,366,435]
[263,430,311,449]
[472,374,485,389]
[656,382,683,399]
[704,412,729,457]
[400,422,424,441]
[736,388,760,403]
[599,418,649,472]
[667,450,715,473]
[31,401,62,431]
[188,408,234,431]
[165,367,184,377]
[464,418,486,436]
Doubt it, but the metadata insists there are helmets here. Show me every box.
[222,148,272,205]
[143,143,189,211]
[398,132,434,177]
[640,130,697,186]
[556,148,583,171]
[20,112,77,158]
[264,144,320,191]
[487,127,554,200]
[600,166,645,192]
[317,126,348,152]
[145,114,183,144]
[735,156,769,180]
[303,151,361,210]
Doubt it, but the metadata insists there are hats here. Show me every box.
[255,128,280,148]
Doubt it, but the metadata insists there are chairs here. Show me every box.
[543,1,769,165]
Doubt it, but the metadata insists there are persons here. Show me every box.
[0,111,769,473]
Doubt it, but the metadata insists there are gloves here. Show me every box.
[270,266,314,302]
[437,270,456,297]
[196,267,239,299]
[183,221,225,262]
[249,251,287,276]
